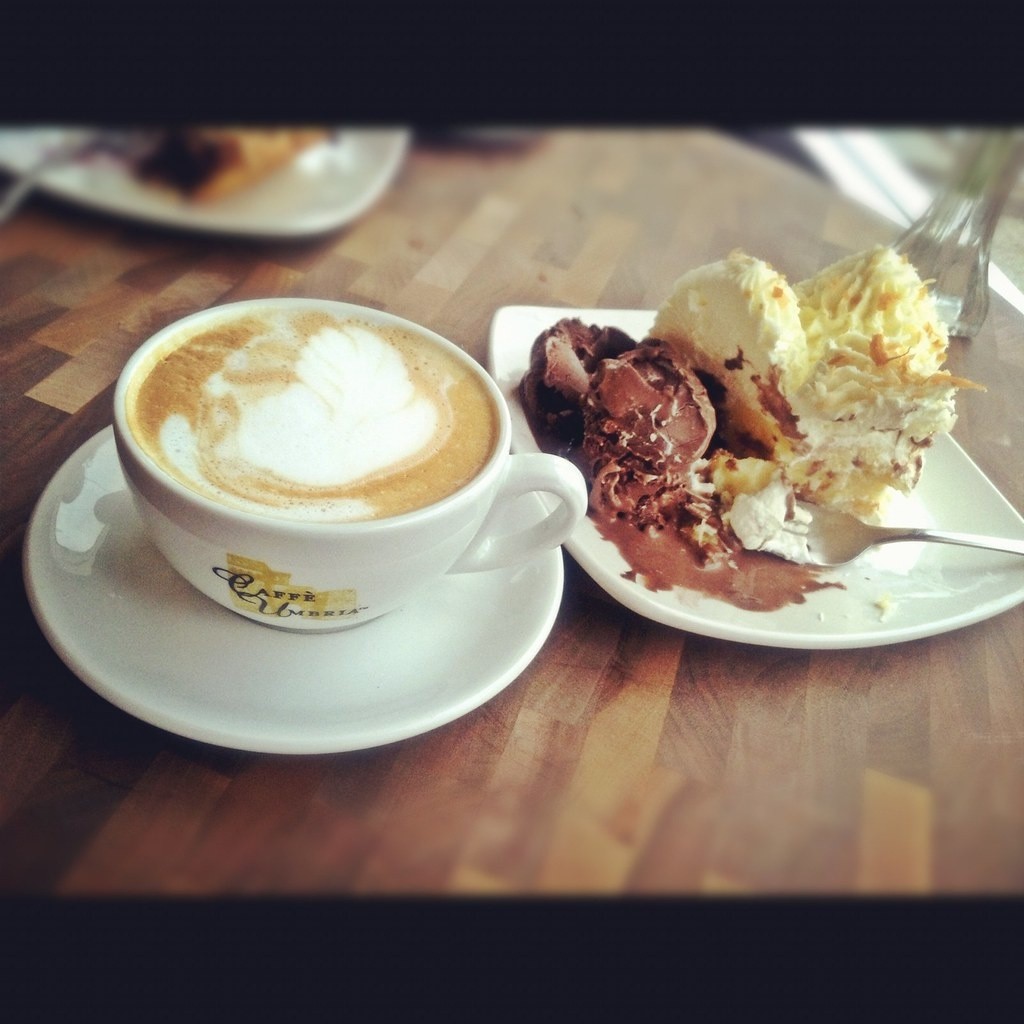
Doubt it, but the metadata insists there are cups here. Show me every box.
[112,294,586,629]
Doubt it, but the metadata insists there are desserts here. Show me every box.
[529,248,958,568]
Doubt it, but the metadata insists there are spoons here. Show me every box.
[733,496,1024,571]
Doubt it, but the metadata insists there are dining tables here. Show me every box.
[0,121,1024,902]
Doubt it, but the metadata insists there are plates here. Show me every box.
[484,298,1024,651]
[24,422,566,756]
[1,122,416,241]
